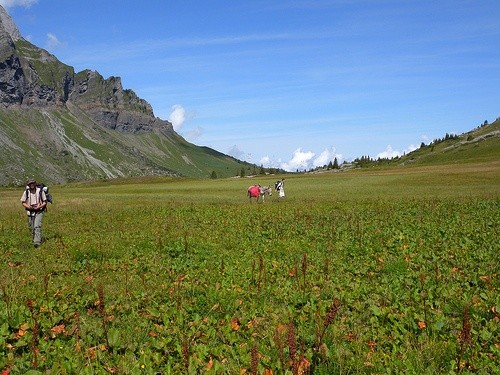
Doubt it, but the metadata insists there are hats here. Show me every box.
[27,179,36,185]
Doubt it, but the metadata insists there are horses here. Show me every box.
[246,184,272,203]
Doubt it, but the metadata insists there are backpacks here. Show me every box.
[25,183,52,204]
[275,181,282,191]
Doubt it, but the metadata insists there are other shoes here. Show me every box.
[34,244,38,248]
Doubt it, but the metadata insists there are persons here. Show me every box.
[20,177,48,248]
[258,183,265,203]
[275,177,286,203]
